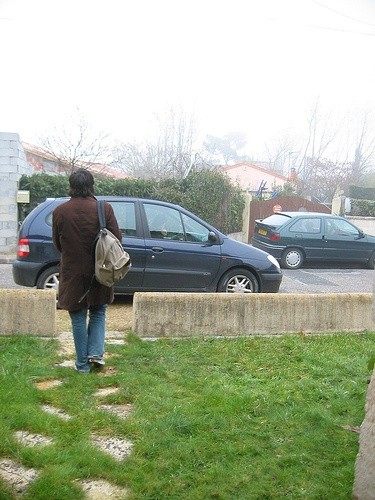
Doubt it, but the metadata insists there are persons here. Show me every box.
[53,168,123,374]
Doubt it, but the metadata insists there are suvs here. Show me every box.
[13,195,284,309]
[251,212,375,270]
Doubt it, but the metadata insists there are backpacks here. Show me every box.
[91,200,132,287]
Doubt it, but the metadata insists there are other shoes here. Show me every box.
[74,365,90,373]
[89,359,106,364]
